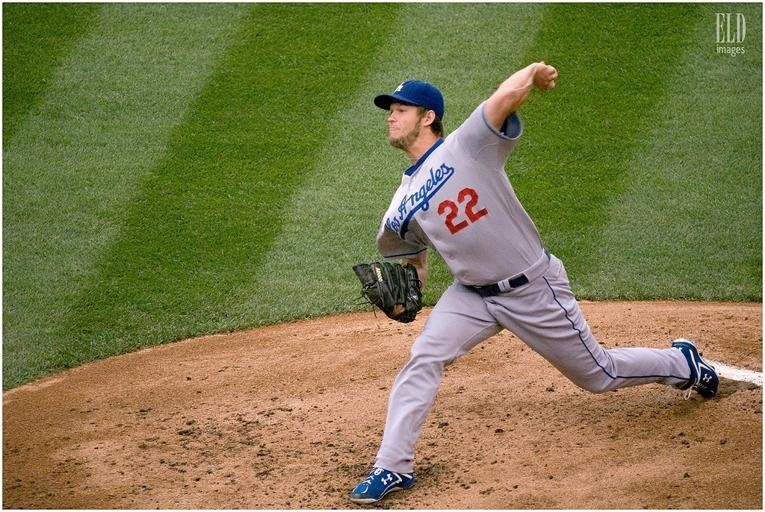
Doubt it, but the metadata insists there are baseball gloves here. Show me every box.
[352,261,422,323]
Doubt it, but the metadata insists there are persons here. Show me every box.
[352,61,720,505]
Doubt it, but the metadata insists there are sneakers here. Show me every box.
[349,464,414,503]
[673,336,720,400]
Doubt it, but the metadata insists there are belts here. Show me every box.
[460,273,528,299]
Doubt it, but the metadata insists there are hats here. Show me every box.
[372,77,444,124]
